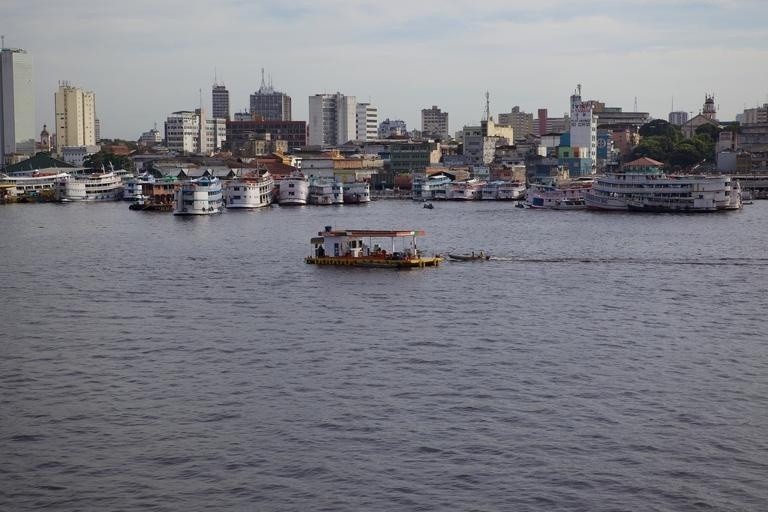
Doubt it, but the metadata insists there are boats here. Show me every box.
[449,250,491,261]
[304,224,444,270]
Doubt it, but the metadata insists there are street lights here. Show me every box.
[381,180,387,194]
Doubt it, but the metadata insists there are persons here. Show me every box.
[400,252,412,261]
[377,248,382,256]
[478,252,483,258]
[471,251,475,258]
